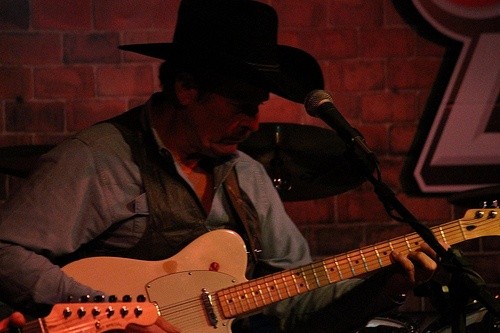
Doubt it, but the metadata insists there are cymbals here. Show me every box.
[240,122,374,201]
[448,185,499,208]
[0,145,60,178]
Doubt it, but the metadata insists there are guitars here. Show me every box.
[57,201,500,333]
[0,293,161,333]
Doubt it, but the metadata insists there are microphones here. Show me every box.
[303,90,379,169]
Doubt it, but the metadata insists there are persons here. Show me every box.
[0,0,451,333]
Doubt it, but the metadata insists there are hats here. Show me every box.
[119,0,324,103]
